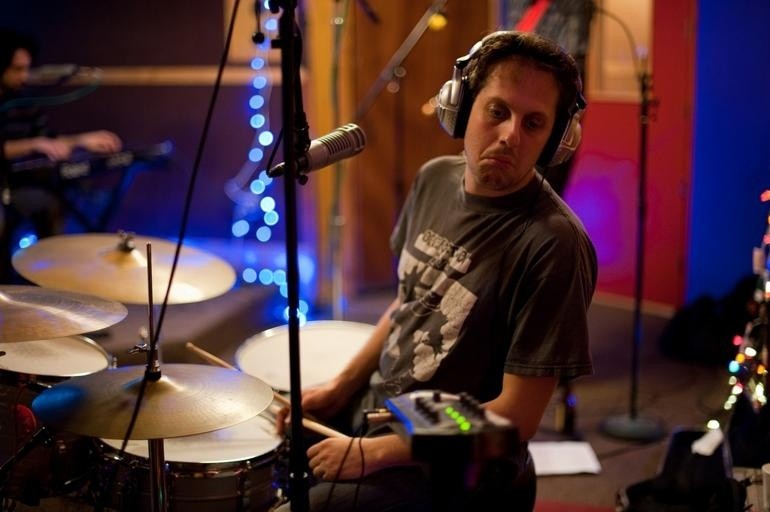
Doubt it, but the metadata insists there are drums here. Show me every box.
[233,320,376,421]
[62,417,291,512]
[0,335,118,506]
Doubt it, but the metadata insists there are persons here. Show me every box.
[0,47,122,239]
[277,30,597,512]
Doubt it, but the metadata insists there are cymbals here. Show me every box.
[32,362,276,441]
[0,285,128,344]
[11,232,237,306]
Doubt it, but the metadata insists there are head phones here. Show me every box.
[435,30,588,168]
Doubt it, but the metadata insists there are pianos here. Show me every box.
[6,139,174,190]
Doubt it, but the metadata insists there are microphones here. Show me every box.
[268,123,368,178]
